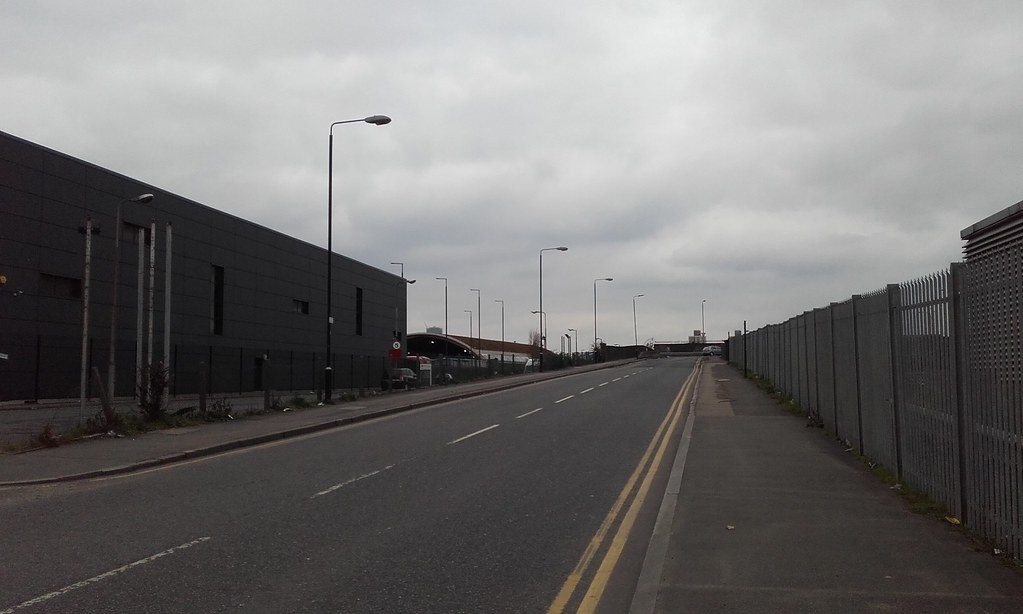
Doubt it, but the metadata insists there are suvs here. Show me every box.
[701,346,722,356]
[380,367,416,391]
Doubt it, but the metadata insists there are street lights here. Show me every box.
[701,300,706,342]
[324,115,391,405]
[569,329,578,354]
[495,299,505,377]
[107,192,154,422]
[471,288,481,368]
[391,262,403,276]
[531,310,548,349]
[463,309,472,355]
[594,278,613,365]
[539,247,569,373]
[633,294,644,360]
[436,277,448,368]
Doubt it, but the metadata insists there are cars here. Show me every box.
[406,333,484,360]
